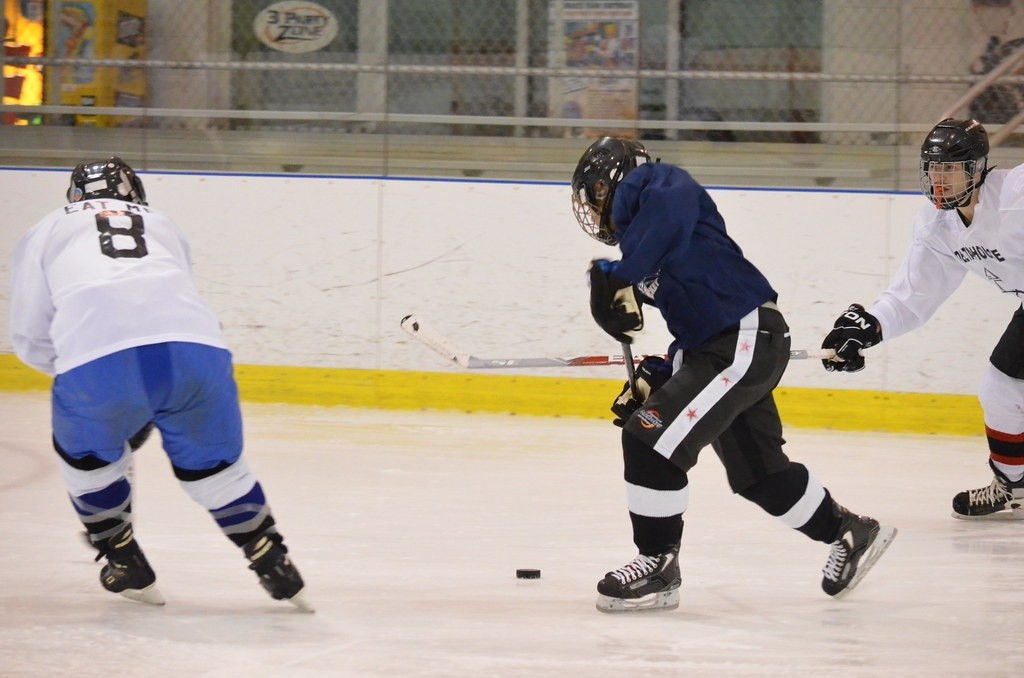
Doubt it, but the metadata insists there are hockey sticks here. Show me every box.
[620,340,645,406]
[399,312,845,370]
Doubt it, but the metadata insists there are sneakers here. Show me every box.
[822,487,898,599]
[246,534,315,612]
[595,520,684,612]
[100,523,165,605]
[951,475,1024,520]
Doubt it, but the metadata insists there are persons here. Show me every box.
[822,117,1024,522]
[11,156,315,615]
[570,136,897,613]
[568,23,618,64]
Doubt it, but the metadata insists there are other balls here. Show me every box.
[515,568,542,580]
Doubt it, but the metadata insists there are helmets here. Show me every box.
[919,118,989,210]
[571,136,651,246]
[66,156,149,206]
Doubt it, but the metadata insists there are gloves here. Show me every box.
[821,303,882,373]
[591,259,640,343]
[611,356,666,428]
